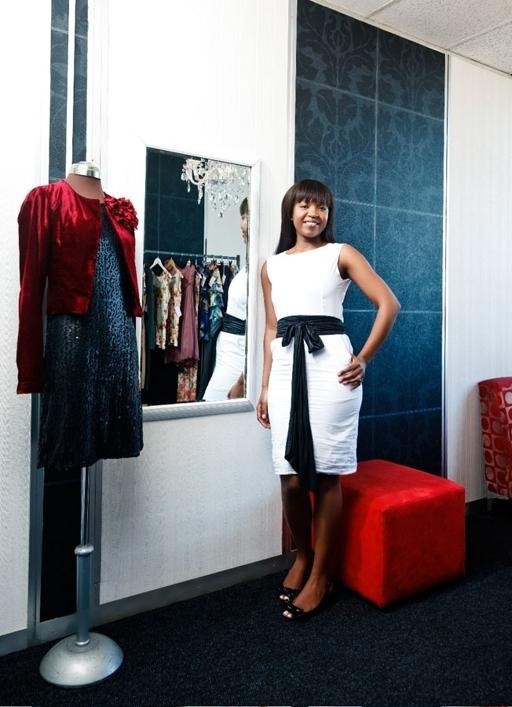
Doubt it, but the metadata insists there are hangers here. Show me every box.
[149,250,184,279]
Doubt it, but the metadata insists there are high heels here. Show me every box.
[277,569,337,621]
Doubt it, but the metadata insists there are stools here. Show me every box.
[304,457,466,609]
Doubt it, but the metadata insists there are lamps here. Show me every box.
[182,153,251,219]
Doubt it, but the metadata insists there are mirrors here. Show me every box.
[138,143,262,428]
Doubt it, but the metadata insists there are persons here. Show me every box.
[203,197,248,401]
[16,162,146,470]
[256,179,401,620]
